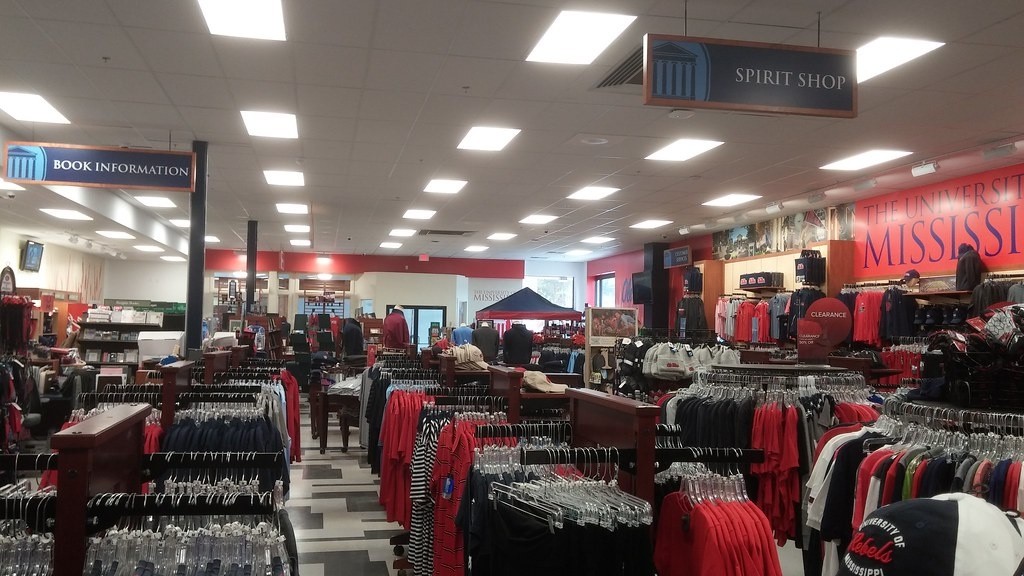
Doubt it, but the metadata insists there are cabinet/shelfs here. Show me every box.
[901,290,974,331]
[76,320,163,365]
[29,310,59,345]
[228,314,283,359]
[360,317,384,355]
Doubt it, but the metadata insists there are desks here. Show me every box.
[32,358,60,379]
[309,380,366,454]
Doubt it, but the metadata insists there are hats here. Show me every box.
[901,269,920,282]
[441,342,489,370]
[834,492,1024,576]
[958,243,971,259]
[523,370,568,393]
[829,347,889,370]
[914,304,967,324]
[617,338,740,381]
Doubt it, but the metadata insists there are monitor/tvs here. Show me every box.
[21,240,44,272]
[631,270,654,305]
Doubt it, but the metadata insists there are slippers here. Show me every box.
[593,352,617,393]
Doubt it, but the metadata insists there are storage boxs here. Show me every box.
[739,271,785,287]
[86,308,112,322]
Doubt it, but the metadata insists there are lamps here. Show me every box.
[910,159,940,177]
[765,202,784,215]
[678,226,690,236]
[808,189,827,204]
[983,138,1016,160]
[854,177,877,192]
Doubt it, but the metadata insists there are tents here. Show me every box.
[475,287,582,338]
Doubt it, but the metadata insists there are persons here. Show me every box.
[591,310,636,339]
[328,312,339,337]
[451,321,534,365]
[383,305,411,349]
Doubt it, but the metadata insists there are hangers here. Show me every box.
[655,422,751,510]
[982,274,1014,286]
[375,355,655,533]
[841,280,901,292]
[883,335,930,354]
[0,360,293,576]
[542,342,572,355]
[729,290,794,304]
[749,342,799,360]
[677,367,875,409]
[800,282,822,293]
[683,289,699,299]
[870,377,1024,465]
